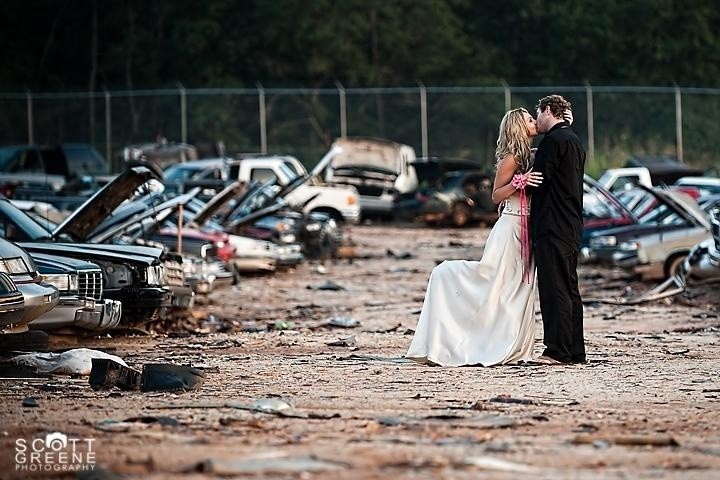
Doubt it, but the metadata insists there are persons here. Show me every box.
[405,107,574,368]
[515,94,586,367]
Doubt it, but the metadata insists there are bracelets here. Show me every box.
[511,173,528,190]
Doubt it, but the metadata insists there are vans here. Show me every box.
[326,138,419,215]
